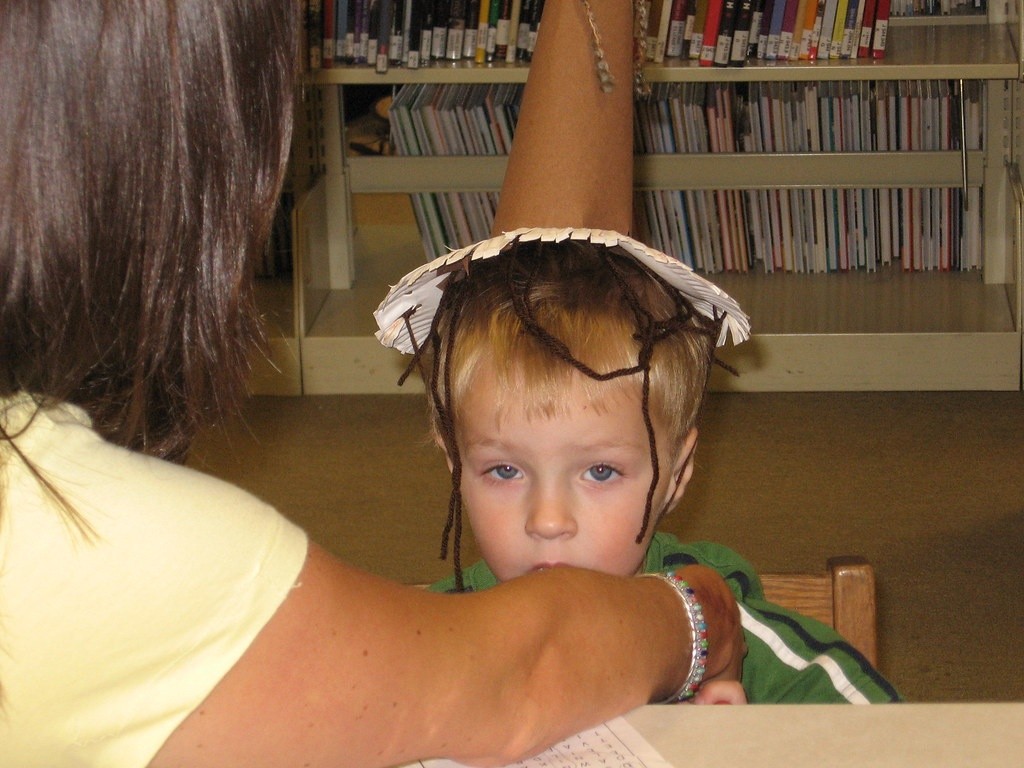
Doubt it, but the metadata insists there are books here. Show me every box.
[303,0,986,75]
[386,186,987,281]
[387,80,983,157]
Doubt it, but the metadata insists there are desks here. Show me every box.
[619,702,1024,768]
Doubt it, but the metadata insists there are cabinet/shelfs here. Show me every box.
[216,1,1024,397]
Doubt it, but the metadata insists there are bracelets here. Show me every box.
[632,570,710,706]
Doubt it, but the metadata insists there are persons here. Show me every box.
[0,0,750,768]
[371,224,907,705]
